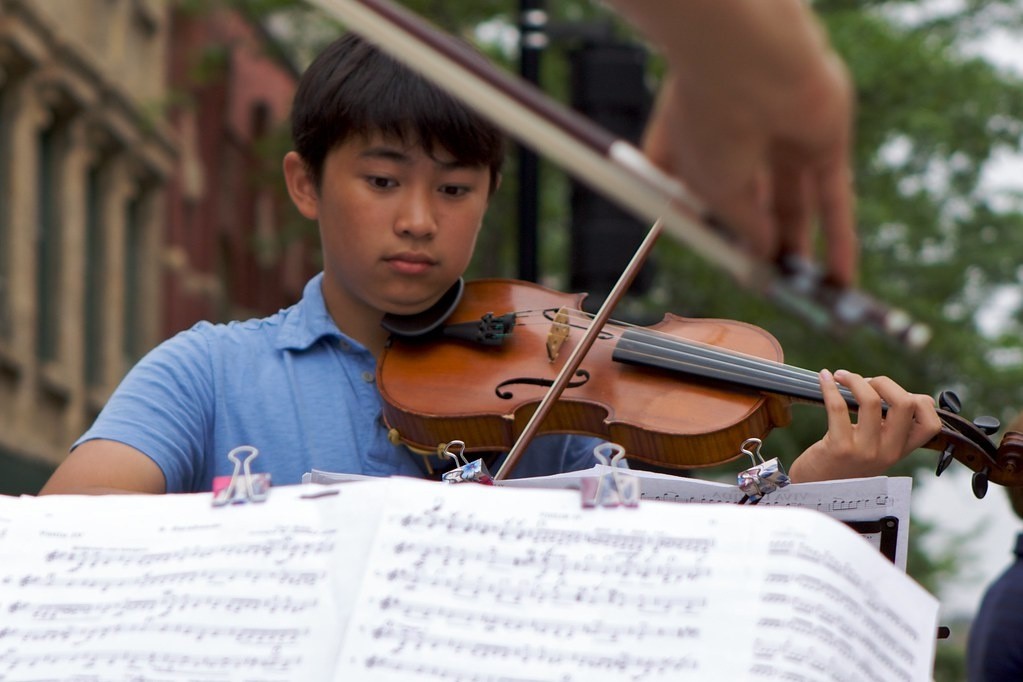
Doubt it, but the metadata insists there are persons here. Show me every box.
[36,31,941,495]
[967,410,1023,682]
[600,0,858,291]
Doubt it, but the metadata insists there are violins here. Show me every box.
[373,276,1023,498]
